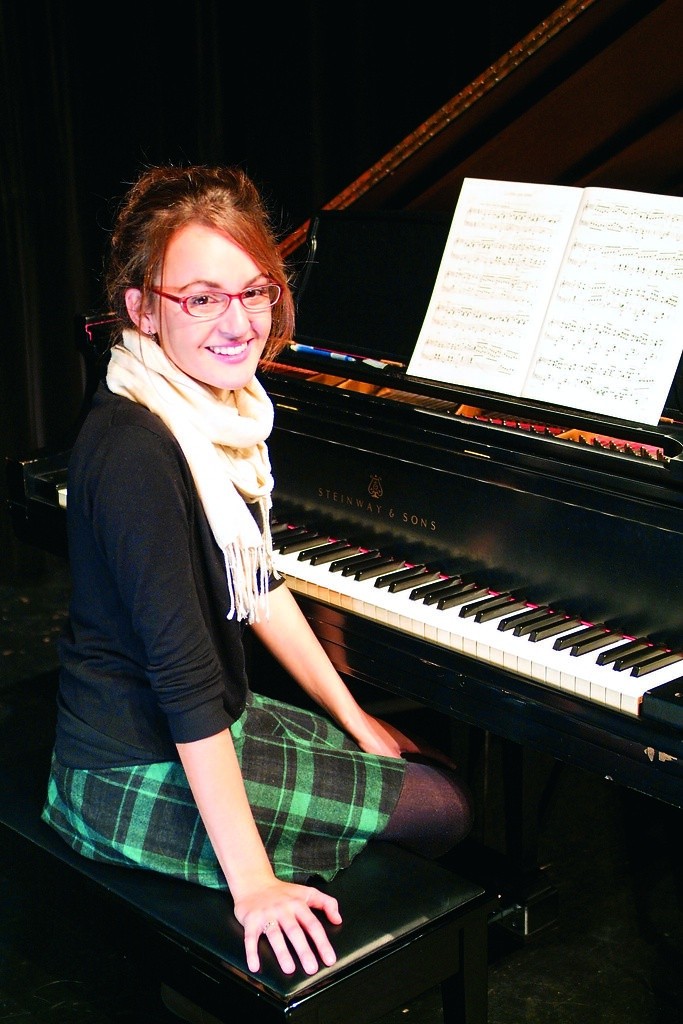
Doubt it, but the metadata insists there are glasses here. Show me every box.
[140,276,283,319]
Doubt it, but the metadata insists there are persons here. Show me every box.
[41,163,477,976]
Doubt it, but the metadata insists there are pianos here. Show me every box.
[5,1,683,1023]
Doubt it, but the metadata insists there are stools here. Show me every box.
[0,664,504,1023]
